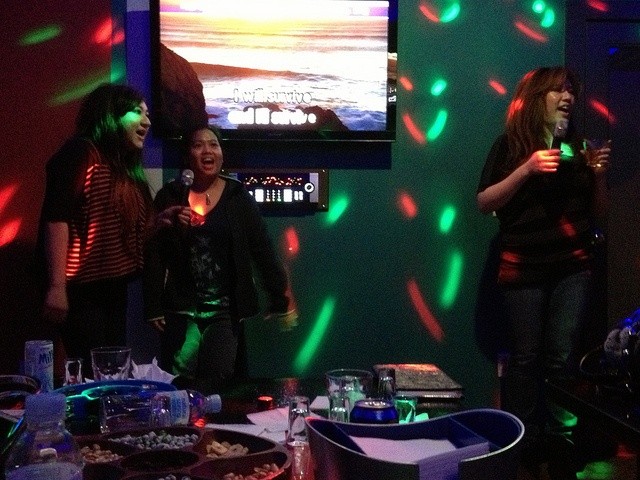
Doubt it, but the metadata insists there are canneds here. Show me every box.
[350,397,399,424]
[23,339,54,393]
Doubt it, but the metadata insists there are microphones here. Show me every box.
[178,170,194,227]
[551,121,568,151]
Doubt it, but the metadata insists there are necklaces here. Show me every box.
[193,175,218,205]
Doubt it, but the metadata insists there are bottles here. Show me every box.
[99,389,221,436]
[5,393,83,480]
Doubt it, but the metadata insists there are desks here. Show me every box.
[192,367,640,480]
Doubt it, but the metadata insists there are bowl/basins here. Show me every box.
[0,375,42,394]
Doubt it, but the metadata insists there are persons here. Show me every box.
[476,67,611,480]
[141,122,298,395]
[37,83,192,376]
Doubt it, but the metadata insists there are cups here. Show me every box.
[91,347,131,381]
[65,359,82,387]
[289,397,310,444]
[330,396,349,423]
[378,369,395,403]
[326,370,373,396]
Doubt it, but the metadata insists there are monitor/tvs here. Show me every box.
[149,0,398,141]
